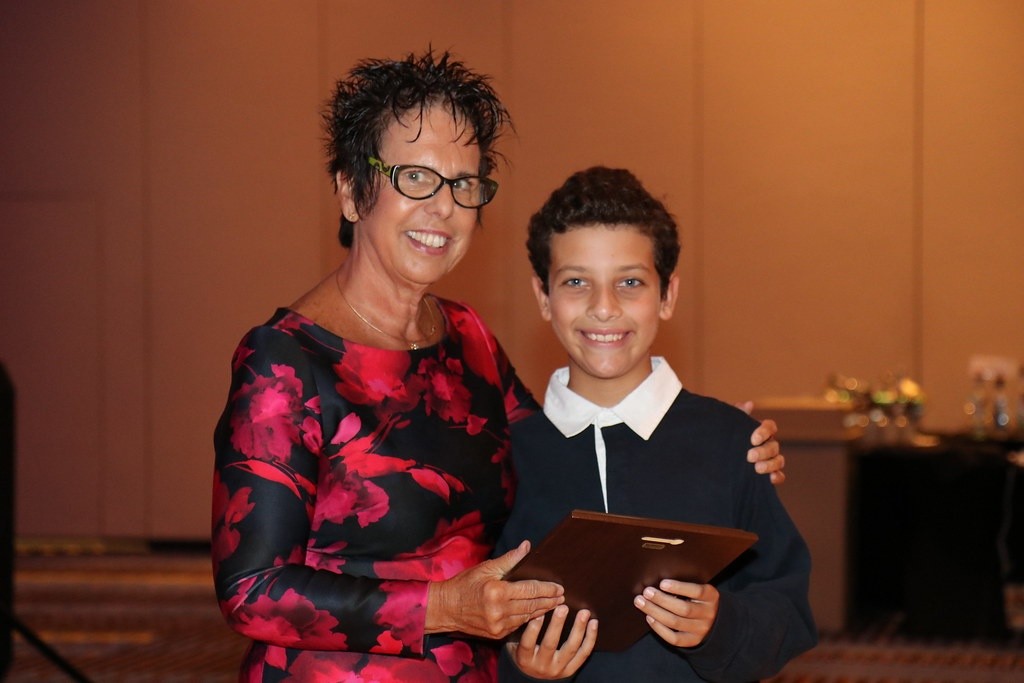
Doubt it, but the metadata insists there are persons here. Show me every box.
[208,48,787,683]
[487,163,821,683]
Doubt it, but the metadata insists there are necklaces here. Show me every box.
[335,268,437,350]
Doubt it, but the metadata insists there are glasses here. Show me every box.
[367,158,499,209]
